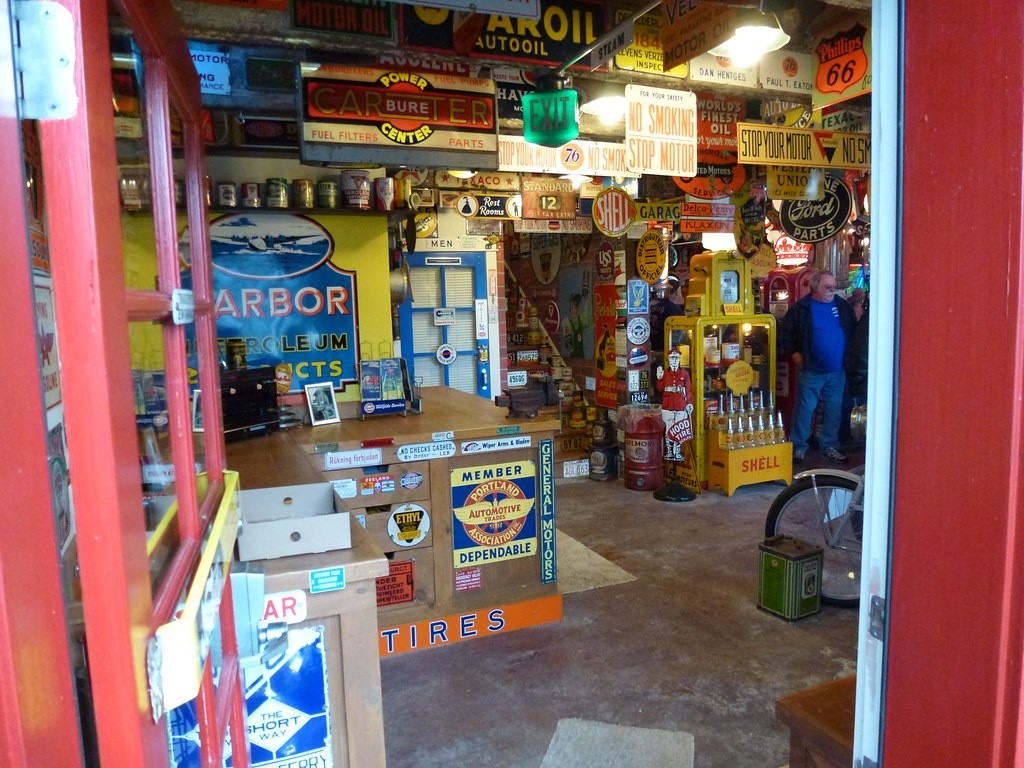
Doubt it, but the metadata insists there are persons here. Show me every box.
[783,270,859,464]
[650,279,684,351]
[839,289,869,447]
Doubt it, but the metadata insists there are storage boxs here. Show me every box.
[233,484,351,563]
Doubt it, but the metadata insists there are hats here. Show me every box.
[668,276,686,294]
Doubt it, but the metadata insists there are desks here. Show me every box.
[147,385,563,768]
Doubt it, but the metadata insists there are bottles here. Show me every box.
[744,337,752,364]
[718,390,787,450]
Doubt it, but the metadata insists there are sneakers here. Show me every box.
[819,447,847,462]
[794,448,807,460]
